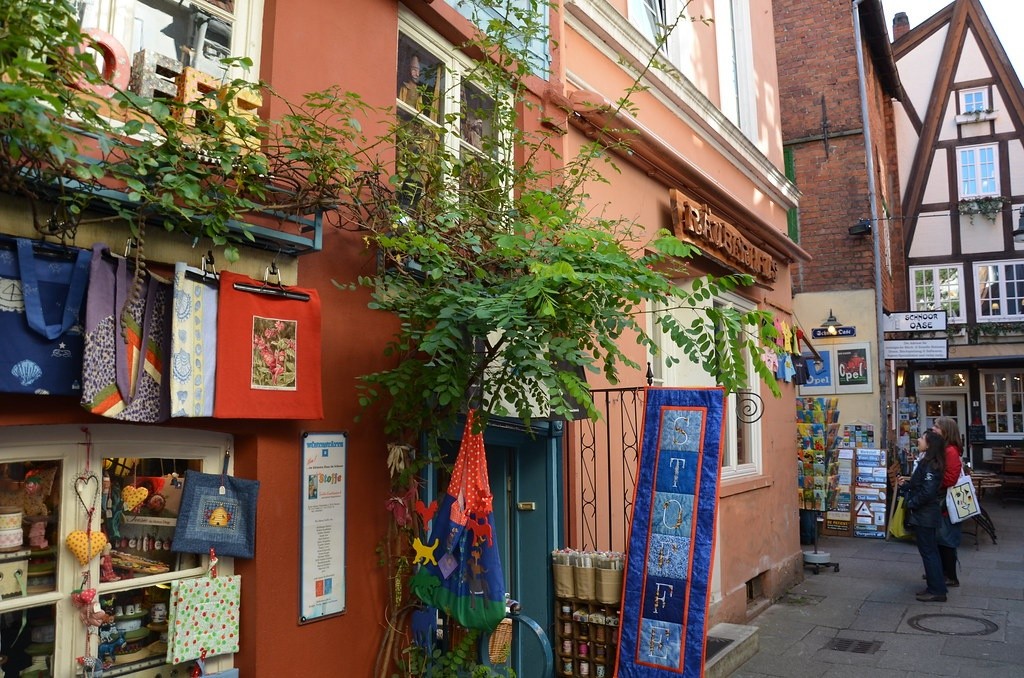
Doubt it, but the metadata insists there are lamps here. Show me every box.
[820,308,843,333]
[992,303,999,315]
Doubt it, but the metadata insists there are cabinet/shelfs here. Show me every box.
[796,397,839,574]
[554,595,621,678]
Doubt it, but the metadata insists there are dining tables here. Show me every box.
[968,471,1004,545]
[1000,450,1024,458]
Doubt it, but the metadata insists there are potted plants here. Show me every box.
[955,110,997,124]
[958,197,1011,225]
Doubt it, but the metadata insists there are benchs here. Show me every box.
[983,447,1024,473]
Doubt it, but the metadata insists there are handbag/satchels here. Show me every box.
[171,451,259,560]
[0,233,323,421]
[945,468,981,525]
[167,560,240,666]
[408,408,505,634]
[889,495,916,539]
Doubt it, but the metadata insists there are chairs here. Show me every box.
[959,478,982,551]
[993,457,1024,509]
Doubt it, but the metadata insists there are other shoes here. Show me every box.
[946,577,960,587]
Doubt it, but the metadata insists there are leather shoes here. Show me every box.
[915,589,948,601]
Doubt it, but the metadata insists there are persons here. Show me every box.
[895,429,950,604]
[920,417,964,588]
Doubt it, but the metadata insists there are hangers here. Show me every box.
[234,243,309,301]
[186,234,220,285]
[109,235,138,271]
[0,196,78,260]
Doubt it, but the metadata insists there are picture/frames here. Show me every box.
[798,344,835,395]
[833,341,873,394]
